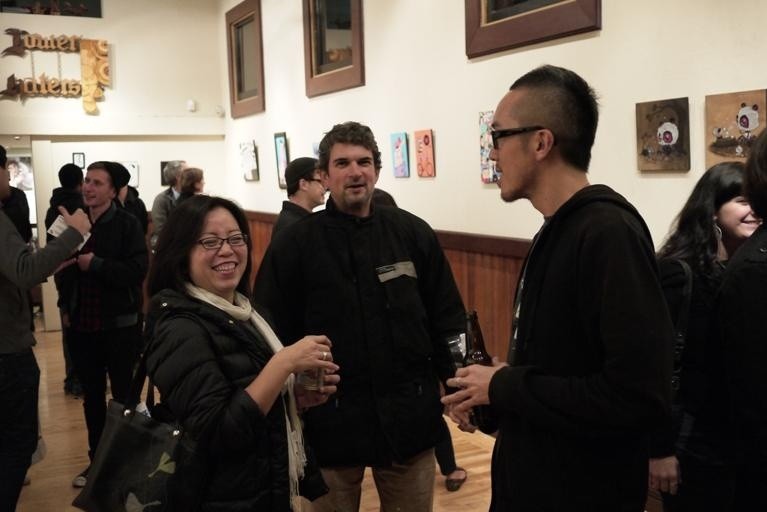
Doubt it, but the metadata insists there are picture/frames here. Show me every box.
[274,132,291,189]
[72,153,85,169]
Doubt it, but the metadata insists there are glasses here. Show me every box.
[197,233,249,250]
[490,126,556,149]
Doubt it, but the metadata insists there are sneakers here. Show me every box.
[73,464,91,488]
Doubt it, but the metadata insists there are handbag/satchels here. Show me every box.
[71,399,183,512]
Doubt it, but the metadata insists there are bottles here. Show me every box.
[463,310,498,425]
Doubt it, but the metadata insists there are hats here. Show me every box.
[59,163,83,188]
[285,157,318,186]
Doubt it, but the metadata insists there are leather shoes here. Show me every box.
[445,466,467,491]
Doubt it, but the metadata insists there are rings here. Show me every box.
[323,351,328,361]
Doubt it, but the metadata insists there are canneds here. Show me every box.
[296,368,325,391]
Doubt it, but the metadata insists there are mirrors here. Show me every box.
[303,0,365,98]
[225,0,265,119]
[465,0,601,58]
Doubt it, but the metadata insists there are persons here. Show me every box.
[712,127,767,512]
[0,144,205,512]
[144,196,341,512]
[371,189,467,491]
[439,66,678,512]
[251,123,468,512]
[646,162,765,512]
[270,157,327,239]
